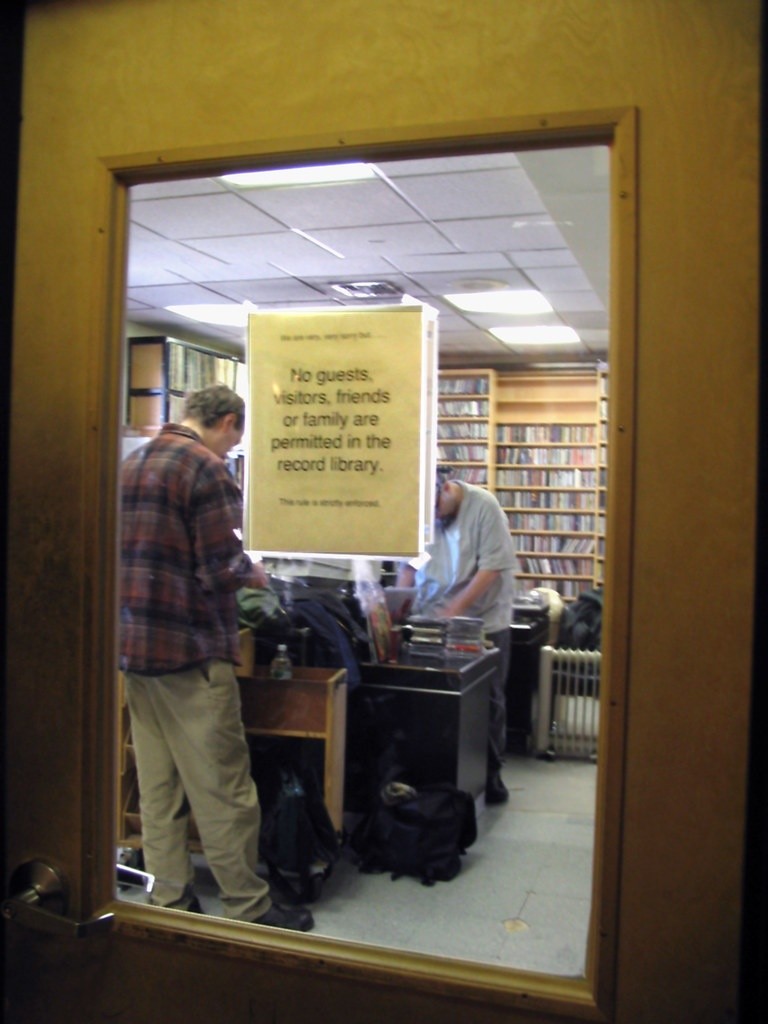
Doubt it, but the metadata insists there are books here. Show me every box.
[169,340,246,490]
[436,370,607,598]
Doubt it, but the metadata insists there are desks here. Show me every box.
[115,600,550,893]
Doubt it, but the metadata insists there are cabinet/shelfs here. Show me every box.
[126,336,610,610]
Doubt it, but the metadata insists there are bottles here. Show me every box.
[270,644,293,680]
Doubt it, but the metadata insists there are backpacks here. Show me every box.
[349,768,478,885]
[557,592,603,652]
[258,764,338,906]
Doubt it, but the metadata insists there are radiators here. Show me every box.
[536,645,603,764]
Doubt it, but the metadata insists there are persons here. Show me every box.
[117,383,316,934]
[413,463,519,805]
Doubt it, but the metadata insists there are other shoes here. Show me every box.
[484,779,508,804]
[252,903,315,933]
